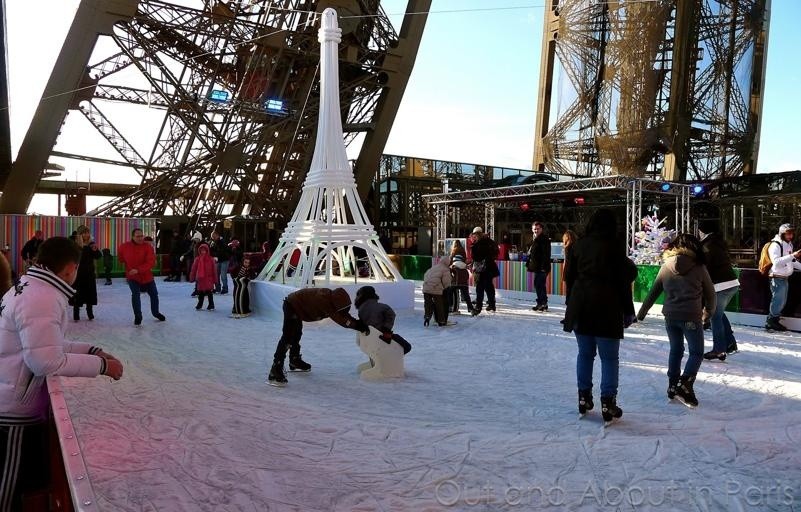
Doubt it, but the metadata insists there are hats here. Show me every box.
[194,231,202,242]
[453,255,463,262]
[473,227,482,234]
[77,225,89,235]
[779,223,795,240]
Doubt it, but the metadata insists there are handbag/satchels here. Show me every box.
[472,259,487,273]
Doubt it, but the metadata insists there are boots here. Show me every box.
[766,316,787,331]
[86,308,94,319]
[578,384,594,414]
[73,308,80,320]
[667,375,698,406]
[601,396,622,421]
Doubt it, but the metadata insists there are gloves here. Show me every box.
[180,256,184,262]
[355,319,364,331]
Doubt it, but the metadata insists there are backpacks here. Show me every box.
[758,241,783,275]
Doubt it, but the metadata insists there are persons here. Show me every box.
[699,220,741,358]
[528,222,551,311]
[560,209,638,420]
[639,233,717,407]
[422,226,499,327]
[1,224,269,512]
[355,285,411,354]
[268,287,368,384]
[766,223,800,331]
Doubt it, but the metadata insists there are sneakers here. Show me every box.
[196,303,203,309]
[134,312,142,325]
[191,288,228,298]
[703,341,737,360]
[269,371,288,383]
[152,312,165,321]
[533,303,548,311]
[289,360,311,370]
[208,305,215,309]
[424,316,430,327]
[447,299,496,317]
